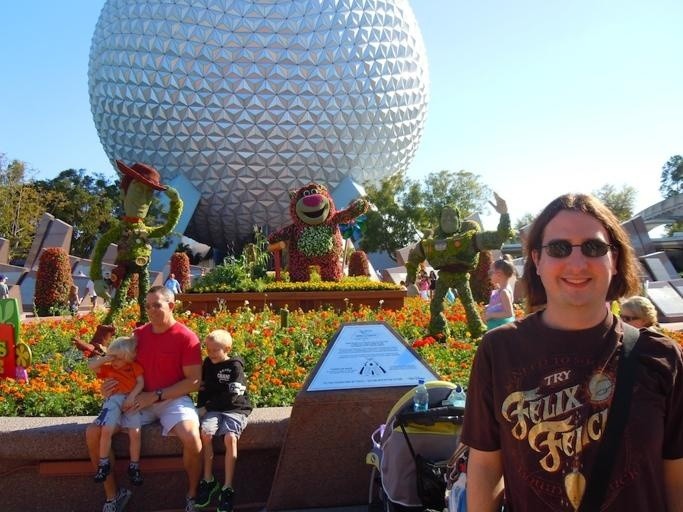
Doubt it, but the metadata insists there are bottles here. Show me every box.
[453,387,467,408]
[414,379,430,411]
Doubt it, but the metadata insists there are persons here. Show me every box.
[80,284,205,512]
[501,253,521,280]
[479,252,522,337]
[78,278,98,310]
[89,334,148,487]
[196,328,255,512]
[73,158,185,356]
[100,271,116,305]
[0,272,10,300]
[617,294,664,332]
[163,272,183,299]
[402,189,513,347]
[396,266,441,301]
[456,193,683,512]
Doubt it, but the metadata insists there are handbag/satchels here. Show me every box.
[416,453,452,508]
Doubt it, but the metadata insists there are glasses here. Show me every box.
[538,239,613,257]
[620,316,640,322]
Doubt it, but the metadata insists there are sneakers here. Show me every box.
[103,489,131,512]
[216,484,234,512]
[193,476,219,507]
[185,496,196,512]
[128,465,144,485]
[94,463,110,481]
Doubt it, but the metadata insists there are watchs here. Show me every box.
[155,388,164,401]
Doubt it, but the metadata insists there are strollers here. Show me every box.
[369,379,469,512]
